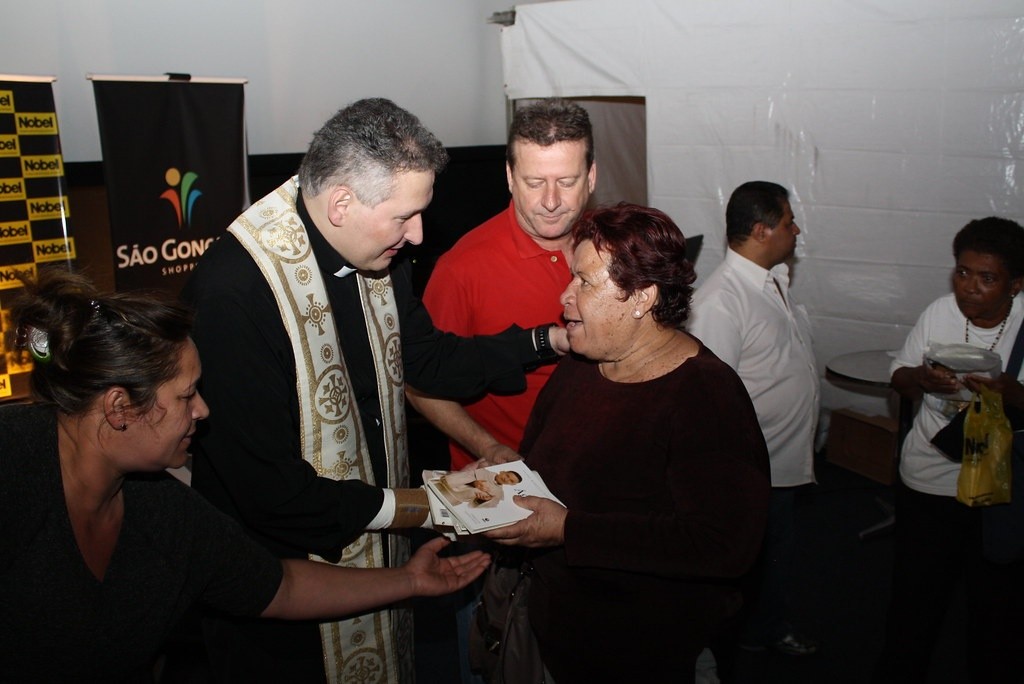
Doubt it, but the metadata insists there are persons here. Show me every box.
[180,99,570,684]
[0,273,492,684]
[404,101,596,683]
[687,181,823,655]
[432,468,523,507]
[869,215,1024,683]
[481,204,771,684]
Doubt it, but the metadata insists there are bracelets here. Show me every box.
[535,322,560,361]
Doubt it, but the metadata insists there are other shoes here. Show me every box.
[739,621,818,654]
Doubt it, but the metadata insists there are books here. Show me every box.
[424,460,567,537]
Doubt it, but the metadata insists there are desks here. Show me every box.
[825,348,914,543]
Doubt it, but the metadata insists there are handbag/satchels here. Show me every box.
[465,545,547,684]
[957,386,1013,506]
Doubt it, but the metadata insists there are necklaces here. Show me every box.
[965,299,1013,351]
